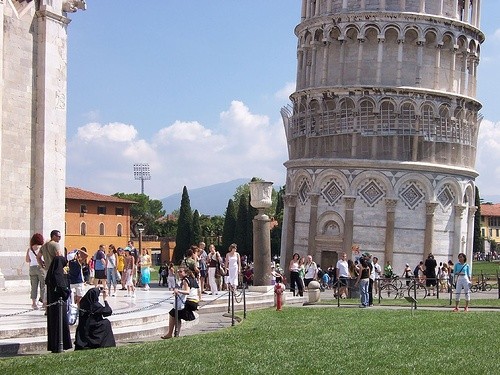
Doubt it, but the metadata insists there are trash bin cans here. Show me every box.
[348,277,360,298]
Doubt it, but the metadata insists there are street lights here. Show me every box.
[133,162,152,194]
[136,222,145,255]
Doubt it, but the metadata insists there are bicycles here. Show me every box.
[379,274,427,300]
[379,270,403,291]
[470,276,492,293]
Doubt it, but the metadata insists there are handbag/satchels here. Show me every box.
[115,270,121,281]
[173,294,187,310]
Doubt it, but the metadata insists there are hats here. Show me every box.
[124,247,131,252]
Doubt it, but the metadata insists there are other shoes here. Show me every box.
[39,298,43,303]
[427,293,430,296]
[124,294,130,297]
[112,293,116,296]
[32,305,39,310]
[119,288,127,290]
[464,306,468,311]
[433,293,436,296]
[161,335,171,339]
[132,293,134,298]
[369,304,373,306]
[359,304,366,307]
[453,308,458,312]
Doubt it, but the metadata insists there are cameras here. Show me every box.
[99,287,104,293]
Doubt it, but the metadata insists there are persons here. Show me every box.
[333,252,381,308]
[104,244,152,297]
[289,252,317,297]
[453,253,471,311]
[271,263,284,285]
[158,261,176,291]
[215,255,254,291]
[473,251,500,262]
[424,252,454,296]
[26,230,62,315]
[67,244,109,304]
[160,264,199,339]
[206,243,223,295]
[225,243,240,295]
[127,240,138,286]
[402,260,425,286]
[384,260,393,282]
[73,288,116,351]
[179,241,208,294]
[316,264,338,294]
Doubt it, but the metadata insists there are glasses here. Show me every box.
[57,236,61,238]
[178,269,185,273]
